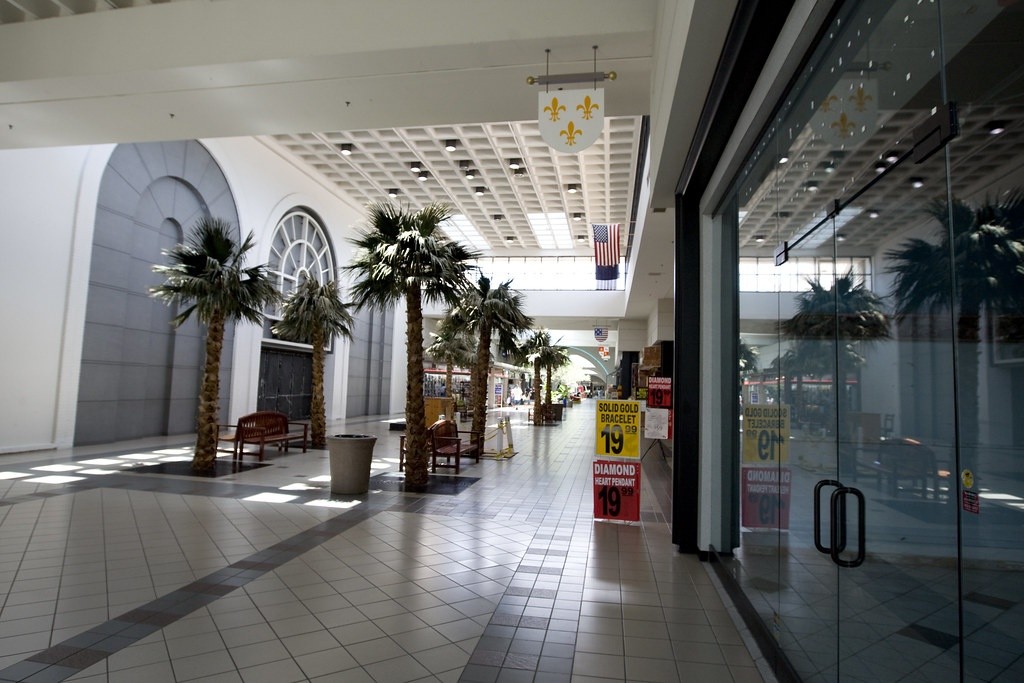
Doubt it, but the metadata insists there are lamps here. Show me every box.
[475,187,485,196]
[419,171,429,181]
[340,144,352,155]
[388,188,399,197]
[506,237,513,241]
[410,162,420,173]
[493,215,501,220]
[514,168,585,243]
[509,159,519,170]
[460,161,470,169]
[445,140,456,152]
[466,170,475,180]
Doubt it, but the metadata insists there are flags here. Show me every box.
[592,224,621,267]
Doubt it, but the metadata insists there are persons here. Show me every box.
[440,383,446,397]
[511,385,523,410]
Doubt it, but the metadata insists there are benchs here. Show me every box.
[528,406,557,425]
[399,419,481,475]
[853,436,951,499]
[215,412,309,462]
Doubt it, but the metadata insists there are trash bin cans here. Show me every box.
[327,434,378,497]
[553,404,562,422]
